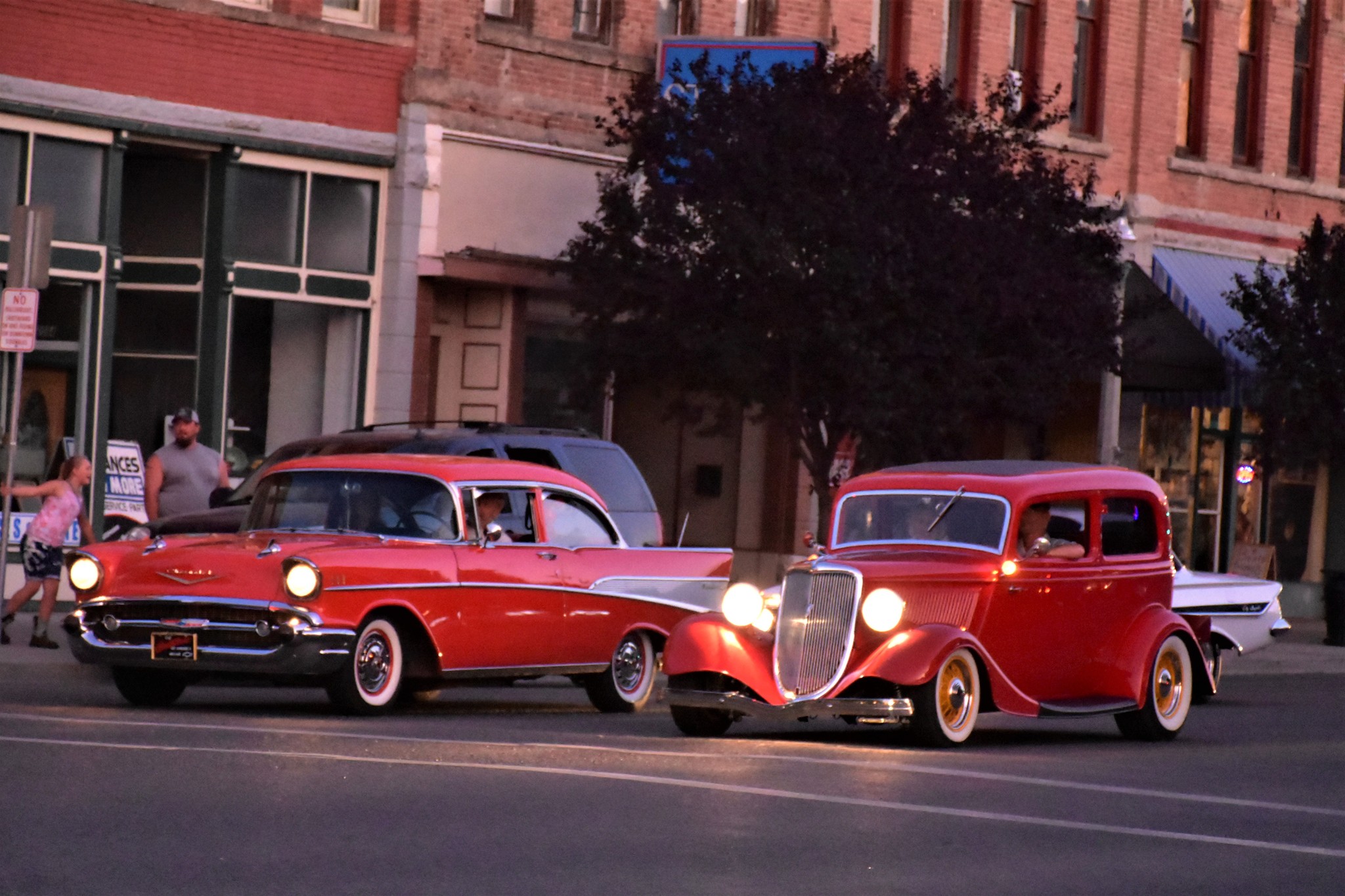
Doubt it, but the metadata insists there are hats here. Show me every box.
[172,407,200,424]
[1029,502,1053,514]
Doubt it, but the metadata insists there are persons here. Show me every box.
[0,454,96,650]
[437,489,513,542]
[143,407,230,522]
[903,502,953,542]
[1019,502,1086,560]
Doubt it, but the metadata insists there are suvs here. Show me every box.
[120,420,665,548]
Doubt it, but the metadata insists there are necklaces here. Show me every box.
[65,480,86,516]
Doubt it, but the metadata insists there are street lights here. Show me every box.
[1093,200,1135,464]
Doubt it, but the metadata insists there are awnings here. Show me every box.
[1123,260,1225,392]
[1152,246,1322,408]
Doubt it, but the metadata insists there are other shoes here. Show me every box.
[0,628,11,644]
[28,635,60,649]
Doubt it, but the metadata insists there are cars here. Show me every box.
[660,459,1293,749]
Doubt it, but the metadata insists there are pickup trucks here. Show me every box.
[60,451,734,718]
